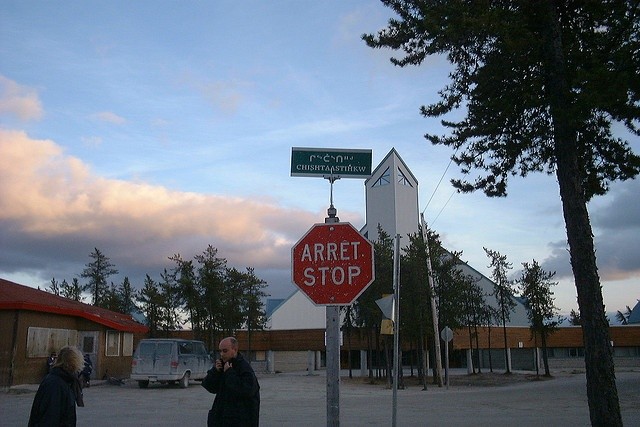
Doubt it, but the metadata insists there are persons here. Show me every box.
[200,336,261,427]
[27,344,90,427]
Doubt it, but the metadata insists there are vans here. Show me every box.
[131,338,215,388]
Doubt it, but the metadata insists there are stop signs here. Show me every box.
[291,221,376,306]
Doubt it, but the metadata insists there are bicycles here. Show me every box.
[101,369,125,386]
